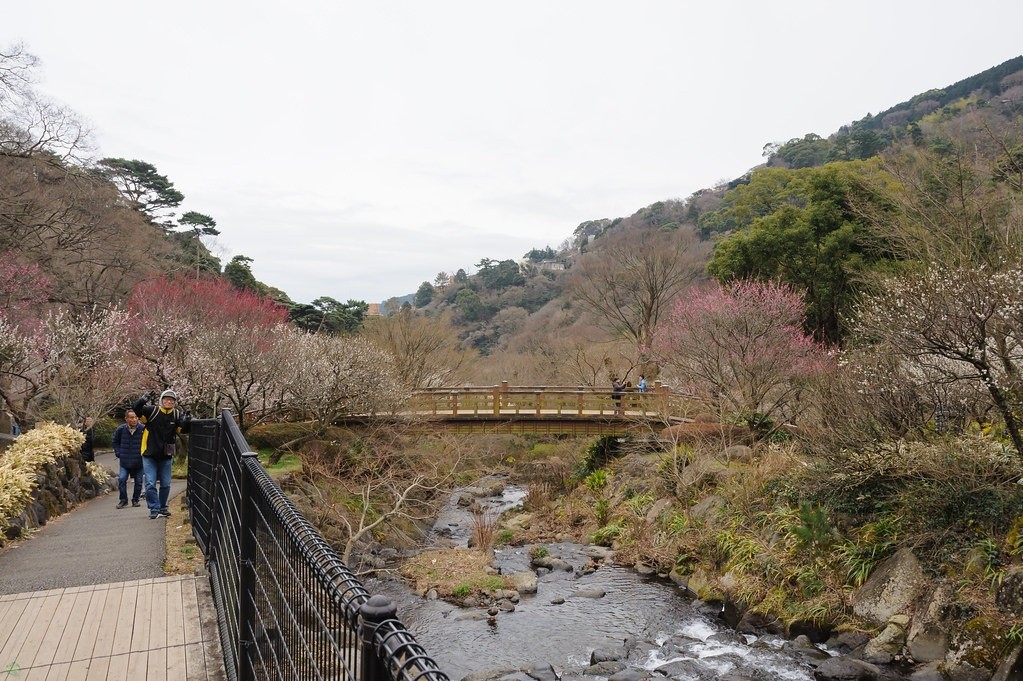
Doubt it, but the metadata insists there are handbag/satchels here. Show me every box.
[163,442,176,458]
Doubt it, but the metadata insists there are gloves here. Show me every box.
[141,389,156,403]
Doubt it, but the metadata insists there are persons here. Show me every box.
[131,388,197,520]
[612,377,627,413]
[78,417,95,463]
[113,409,147,508]
[634,373,649,412]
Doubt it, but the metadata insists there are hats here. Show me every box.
[164,392,176,400]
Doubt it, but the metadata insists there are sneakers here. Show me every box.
[131,500,140,507]
[158,509,171,517]
[148,510,159,520]
[116,500,128,509]
